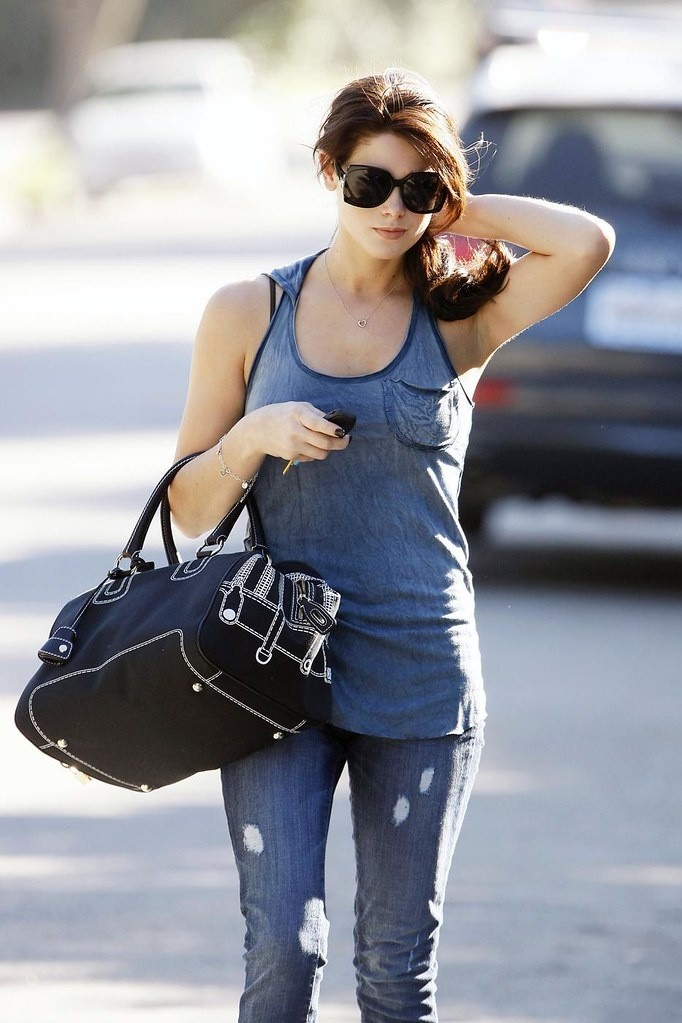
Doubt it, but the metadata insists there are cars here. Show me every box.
[448,26,682,565]
[61,36,271,198]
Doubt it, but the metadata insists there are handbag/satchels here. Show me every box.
[15,452,341,789]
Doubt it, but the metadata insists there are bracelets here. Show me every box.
[217,435,260,503]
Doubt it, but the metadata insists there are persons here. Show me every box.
[166,73,614,1022]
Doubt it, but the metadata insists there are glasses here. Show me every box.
[335,159,448,214]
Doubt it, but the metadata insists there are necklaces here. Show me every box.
[324,249,404,328]
